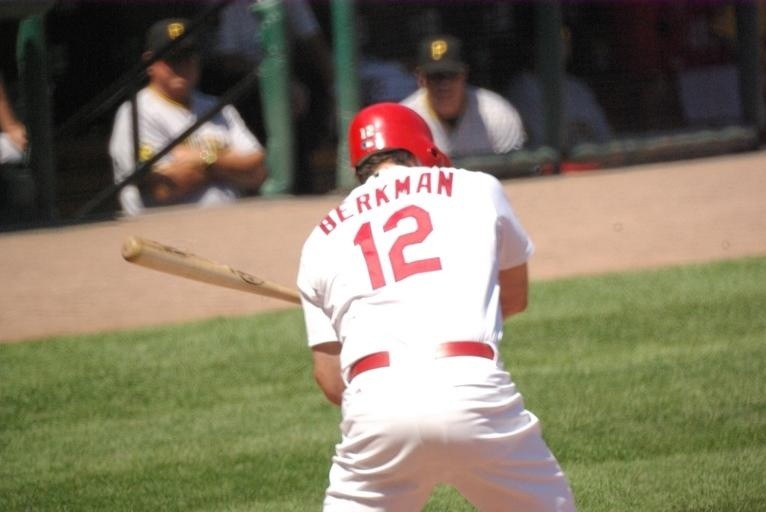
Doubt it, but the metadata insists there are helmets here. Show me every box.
[347,101,450,168]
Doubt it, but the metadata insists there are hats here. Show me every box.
[420,35,468,76]
[145,16,213,51]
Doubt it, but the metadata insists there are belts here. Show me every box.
[347,339,494,384]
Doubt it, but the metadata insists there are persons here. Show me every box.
[212,0,765,189]
[106,17,265,218]
[296,103,578,512]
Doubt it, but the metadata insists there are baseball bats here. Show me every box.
[121,235,302,303]
